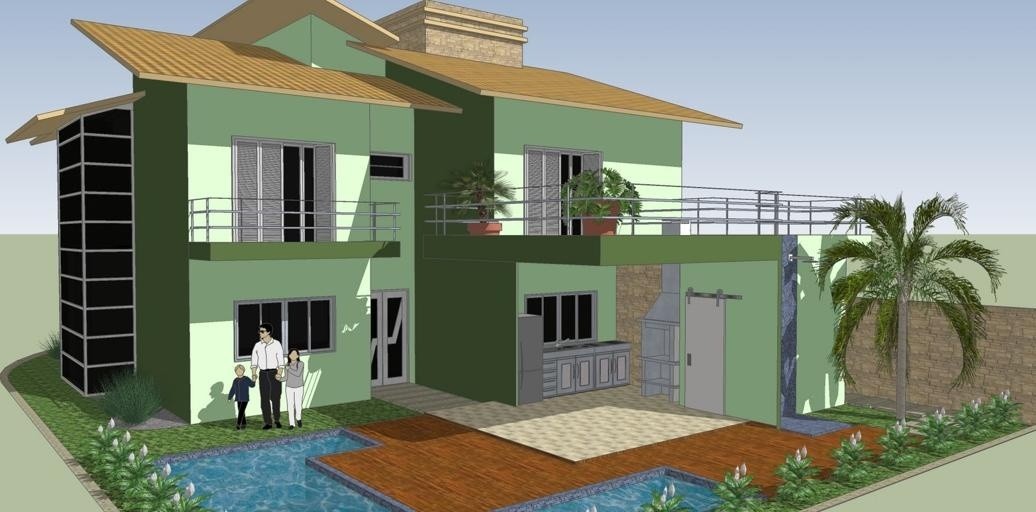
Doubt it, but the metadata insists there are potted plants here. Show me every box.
[442,160,512,236]
[556,168,641,236]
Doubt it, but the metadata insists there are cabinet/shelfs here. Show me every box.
[542,343,631,399]
[638,318,680,403]
